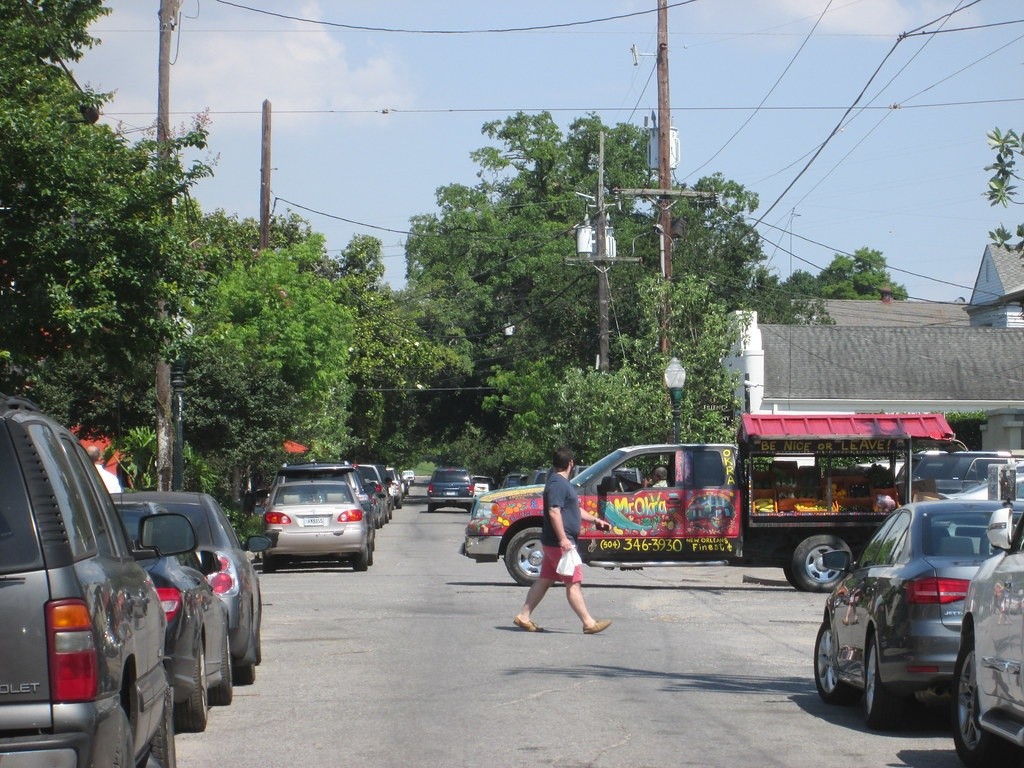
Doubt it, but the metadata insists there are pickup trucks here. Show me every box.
[459,415,969,593]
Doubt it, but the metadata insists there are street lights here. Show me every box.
[664,358,686,445]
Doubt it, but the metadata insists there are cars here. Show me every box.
[428,466,475,513]
[471,465,644,499]
[0,394,274,768]
[254,461,415,571]
[814,449,1024,768]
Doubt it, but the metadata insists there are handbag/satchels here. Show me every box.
[556,545,582,577]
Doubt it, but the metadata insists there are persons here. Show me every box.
[835,577,865,625]
[642,467,668,488]
[96,582,150,638]
[513,448,610,633]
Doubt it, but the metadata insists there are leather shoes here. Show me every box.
[513,617,544,632]
[583,619,613,634]
